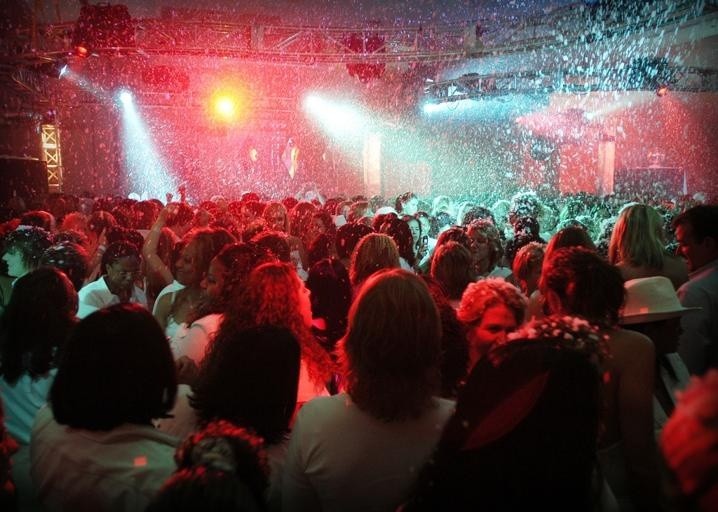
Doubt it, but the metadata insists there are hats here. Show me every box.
[620,276,703,325]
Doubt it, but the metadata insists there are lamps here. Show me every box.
[70,4,136,62]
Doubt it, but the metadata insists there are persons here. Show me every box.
[1,184,718,512]
